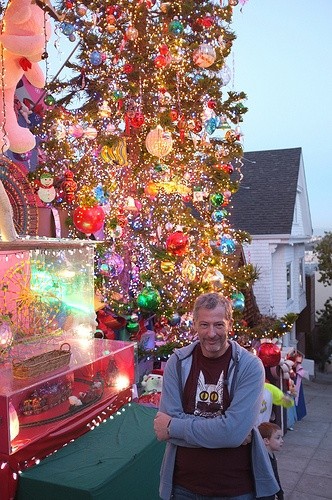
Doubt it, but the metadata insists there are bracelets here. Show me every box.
[167,419,172,435]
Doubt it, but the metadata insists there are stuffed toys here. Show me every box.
[0,0,52,154]
[0,180,18,243]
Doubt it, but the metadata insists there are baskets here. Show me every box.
[10,342,72,379]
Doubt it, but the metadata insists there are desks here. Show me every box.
[13,402,167,500]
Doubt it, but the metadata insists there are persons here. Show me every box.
[152,292,280,500]
[254,380,294,500]
